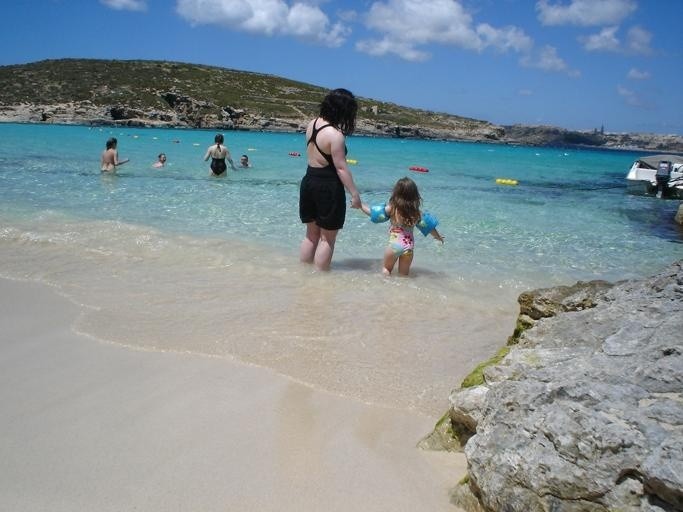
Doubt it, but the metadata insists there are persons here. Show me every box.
[237,155,251,170]
[347,175,446,277]
[150,153,167,169]
[97,137,129,176]
[294,88,361,272]
[203,133,234,178]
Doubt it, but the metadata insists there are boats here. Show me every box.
[624,155,683,200]
